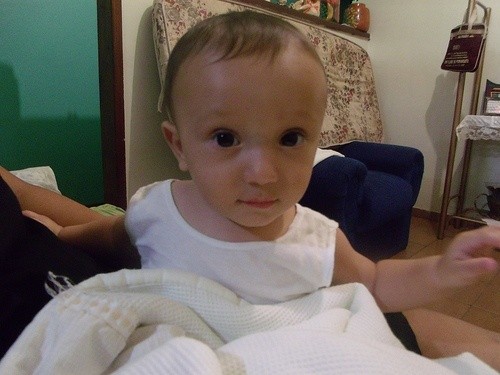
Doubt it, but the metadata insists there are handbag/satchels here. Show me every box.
[441,6,488,73]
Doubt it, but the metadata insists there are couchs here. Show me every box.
[298,142,424,256]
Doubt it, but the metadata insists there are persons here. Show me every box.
[21,11,500,315]
[0,167,500,375]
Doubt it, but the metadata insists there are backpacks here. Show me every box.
[474,186,500,221]
